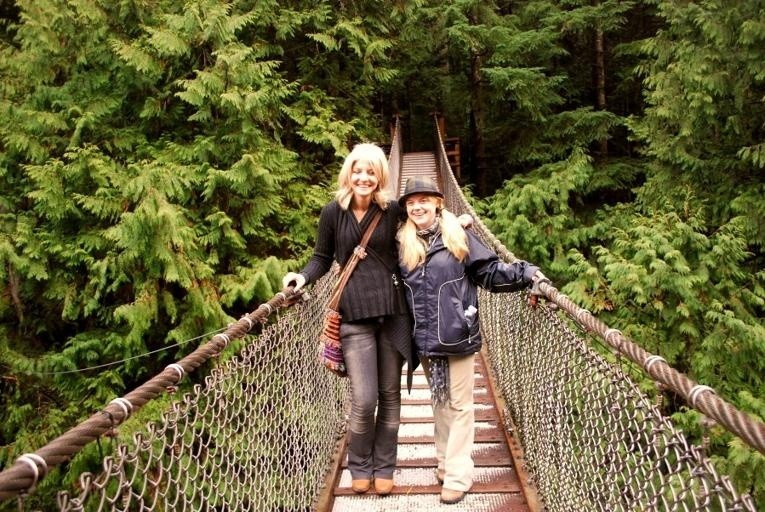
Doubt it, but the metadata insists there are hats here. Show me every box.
[397,176,443,207]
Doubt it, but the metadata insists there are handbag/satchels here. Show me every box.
[323,309,348,376]
[396,287,410,315]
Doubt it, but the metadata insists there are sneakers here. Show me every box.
[437,469,444,480]
[352,480,370,492]
[374,479,393,494]
[439,487,464,504]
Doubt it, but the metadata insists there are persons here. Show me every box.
[395,177,552,505]
[280,144,474,497]
